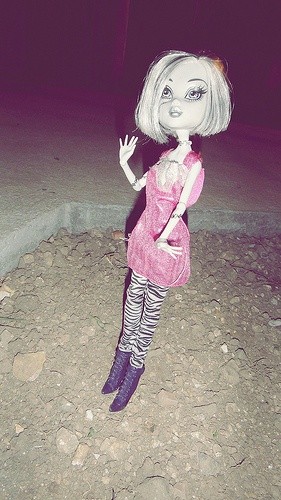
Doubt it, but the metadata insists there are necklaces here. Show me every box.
[179,140,192,145]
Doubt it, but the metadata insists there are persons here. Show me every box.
[101,50,232,411]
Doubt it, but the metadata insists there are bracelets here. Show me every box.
[170,213,182,218]
[131,176,137,187]
